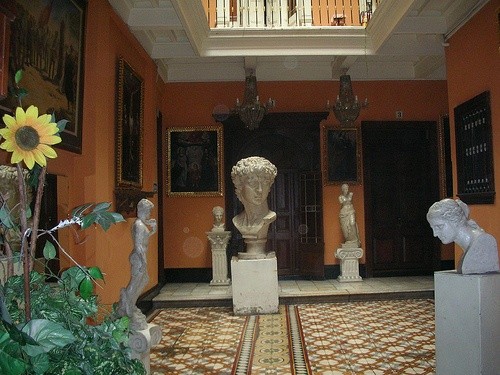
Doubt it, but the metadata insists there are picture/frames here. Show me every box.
[0,0,88,154]
[165,125,226,198]
[438,112,454,199]
[114,57,146,189]
[322,124,362,187]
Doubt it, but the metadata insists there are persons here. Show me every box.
[210,206,226,231]
[426,198,500,274]
[338,184,363,247]
[124,198,157,305]
[232,156,278,260]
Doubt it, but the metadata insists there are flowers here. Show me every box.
[0,68,147,375]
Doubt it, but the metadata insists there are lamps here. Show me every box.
[231,73,275,130]
[324,72,368,127]
